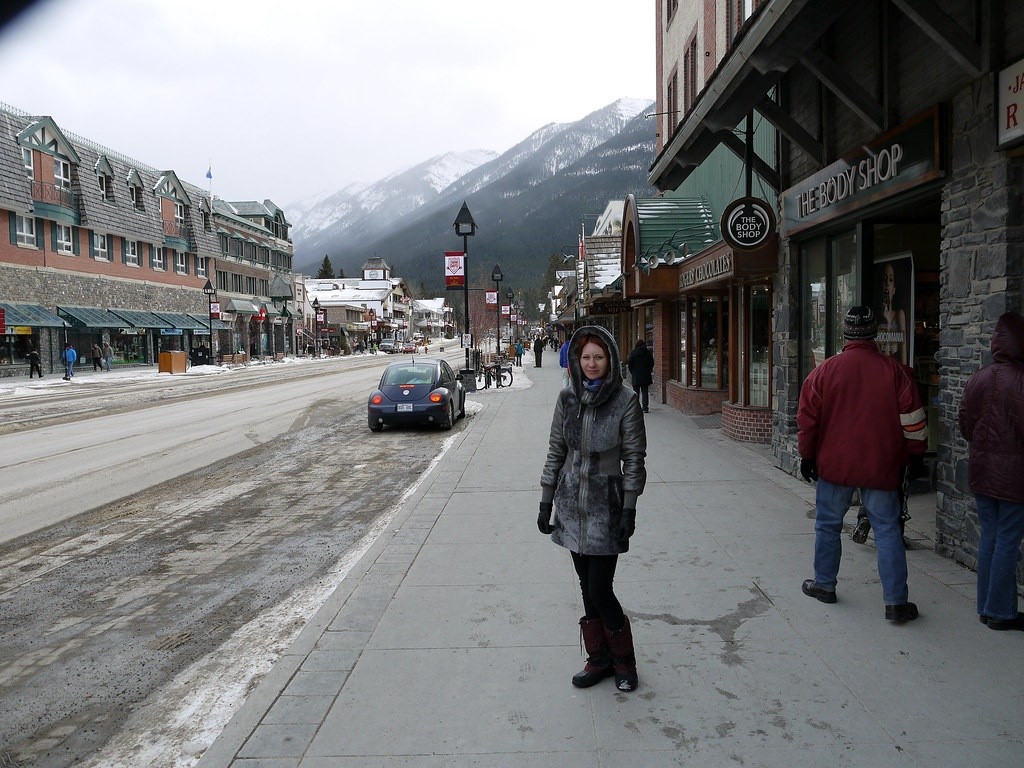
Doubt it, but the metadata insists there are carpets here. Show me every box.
[689,412,725,430]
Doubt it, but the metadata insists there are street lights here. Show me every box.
[369,308,374,347]
[202,278,216,364]
[506,286,515,345]
[513,299,521,339]
[491,263,504,355]
[452,201,478,374]
[312,298,320,352]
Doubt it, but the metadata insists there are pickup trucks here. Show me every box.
[379,339,399,354]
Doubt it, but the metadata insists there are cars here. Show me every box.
[403,343,418,354]
[502,336,510,343]
[368,358,466,432]
[399,343,404,351]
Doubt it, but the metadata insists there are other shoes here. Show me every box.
[39,375,44,378]
[852,517,871,544]
[979,611,1024,631]
[643,407,648,413]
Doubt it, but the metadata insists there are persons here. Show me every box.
[516,339,525,367]
[959,312,1024,631]
[532,332,563,352]
[797,305,929,621]
[537,326,648,679]
[303,335,430,355]
[92,341,114,372]
[29,349,44,378]
[559,333,573,388]
[62,344,76,377]
[852,455,912,548]
[533,333,543,368]
[872,263,908,366]
[628,339,654,410]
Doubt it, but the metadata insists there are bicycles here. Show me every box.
[474,357,513,390]
[186,354,193,370]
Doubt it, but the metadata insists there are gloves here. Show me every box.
[616,509,636,543]
[907,454,925,481]
[800,458,818,483]
[537,501,556,534]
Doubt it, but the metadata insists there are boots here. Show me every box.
[572,616,638,692]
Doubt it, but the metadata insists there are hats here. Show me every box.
[843,306,878,340]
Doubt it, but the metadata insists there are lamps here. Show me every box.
[632,220,720,277]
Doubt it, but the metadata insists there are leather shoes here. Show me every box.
[802,579,837,604]
[885,602,920,623]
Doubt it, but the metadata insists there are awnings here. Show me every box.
[226,300,257,314]
[263,303,280,316]
[0,303,233,329]
[283,305,302,318]
